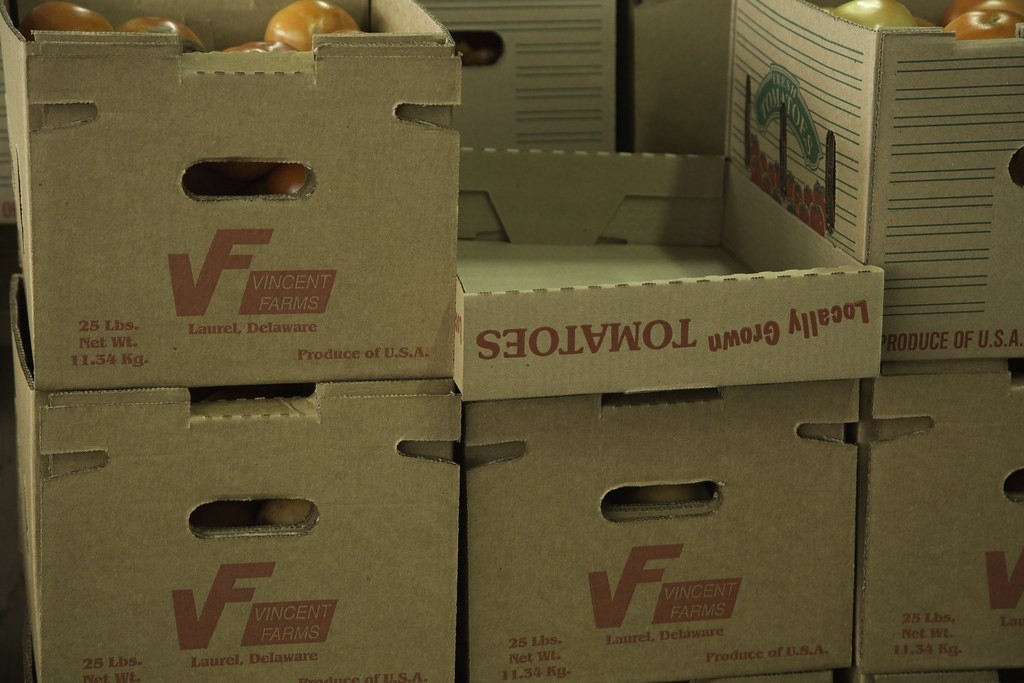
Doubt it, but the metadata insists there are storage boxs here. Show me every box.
[0,0,1024,683]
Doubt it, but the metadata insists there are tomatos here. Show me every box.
[20,0,367,196]
[812,0,1024,42]
